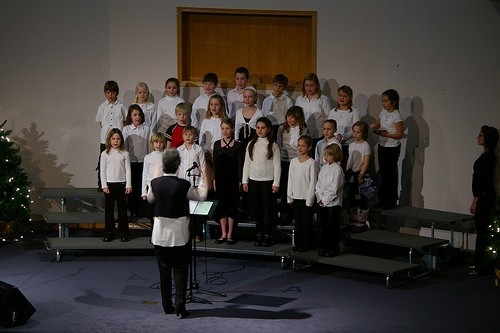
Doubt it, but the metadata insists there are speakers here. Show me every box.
[0,281,37,329]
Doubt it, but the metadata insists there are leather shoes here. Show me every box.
[121,236,128,242]
[104,236,114,242]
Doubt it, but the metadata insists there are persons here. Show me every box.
[369,89,404,210]
[470,125,500,289]
[100,128,132,241]
[96,81,157,223]
[242,117,282,247]
[286,134,317,252]
[315,143,344,258]
[177,125,206,242]
[157,67,371,232]
[212,118,243,245]
[141,132,167,242]
[147,148,207,318]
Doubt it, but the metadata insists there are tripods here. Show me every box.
[171,198,224,306]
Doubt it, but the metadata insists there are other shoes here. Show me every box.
[177,310,190,318]
[165,306,175,314]
[318,246,340,257]
[196,236,202,242]
[215,237,226,244]
[292,244,316,252]
[468,264,479,275]
[255,236,275,246]
[225,238,235,245]
